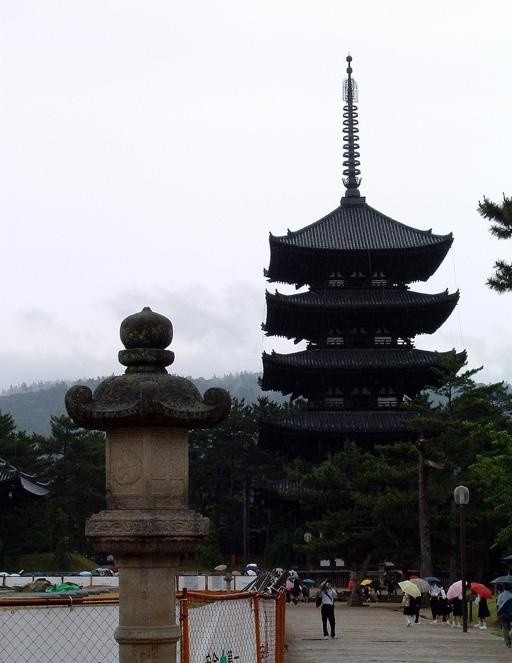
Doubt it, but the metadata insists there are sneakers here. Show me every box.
[429,621,463,628]
[473,624,487,630]
[406,622,422,627]
[325,635,339,641]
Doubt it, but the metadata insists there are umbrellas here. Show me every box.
[214,562,314,605]
[315,577,329,608]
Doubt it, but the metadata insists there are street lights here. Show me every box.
[453,485,471,634]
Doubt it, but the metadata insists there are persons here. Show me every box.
[319,581,337,638]
[496,583,512,648]
[348,573,511,629]
